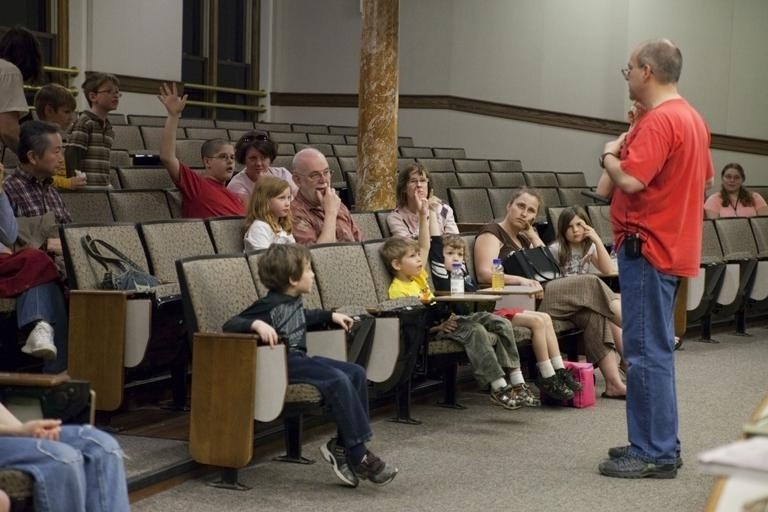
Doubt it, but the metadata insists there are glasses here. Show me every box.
[241,135,269,145]
[295,168,335,180]
[621,68,633,81]
[408,178,429,184]
[208,152,235,161]
[98,89,121,98]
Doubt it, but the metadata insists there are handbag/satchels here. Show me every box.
[102,267,161,292]
[504,246,562,284]
[540,360,596,408]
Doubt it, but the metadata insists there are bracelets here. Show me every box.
[599,152,620,165]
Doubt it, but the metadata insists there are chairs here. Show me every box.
[0,371,98,512]
[173,250,347,493]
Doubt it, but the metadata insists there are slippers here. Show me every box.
[602,390,626,400]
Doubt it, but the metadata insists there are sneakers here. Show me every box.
[555,368,582,391]
[512,383,540,407]
[489,385,524,410]
[599,454,677,478]
[320,438,358,487]
[538,371,574,401]
[349,446,399,487]
[608,446,683,468]
[21,320,58,361]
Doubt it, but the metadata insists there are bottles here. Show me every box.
[450,263,464,296]
[491,258,505,290]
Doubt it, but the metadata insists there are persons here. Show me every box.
[1,25,132,358]
[597,39,714,478]
[246,148,355,252]
[546,206,628,376]
[222,244,398,489]
[0,398,129,510]
[153,79,299,218]
[379,237,542,409]
[703,161,768,218]
[427,194,584,400]
[472,189,681,398]
[387,165,462,235]
